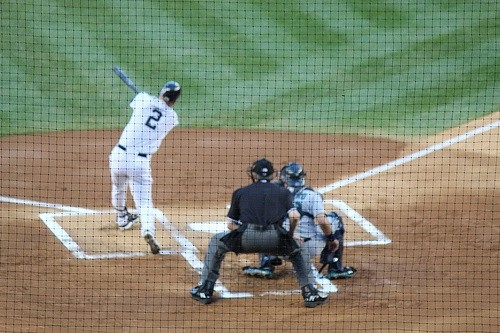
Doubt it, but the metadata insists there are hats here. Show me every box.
[247,159,276,180]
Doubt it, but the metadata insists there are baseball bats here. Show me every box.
[113,67,141,94]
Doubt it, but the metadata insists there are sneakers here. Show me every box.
[190,281,215,305]
[301,285,330,308]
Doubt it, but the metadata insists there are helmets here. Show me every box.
[160,81,182,105]
[279,162,306,187]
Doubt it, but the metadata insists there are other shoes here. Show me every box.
[143,230,161,254]
[328,266,358,279]
[242,260,276,279]
[117,213,140,230]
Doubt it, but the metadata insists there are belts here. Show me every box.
[118,145,147,158]
[247,224,277,231]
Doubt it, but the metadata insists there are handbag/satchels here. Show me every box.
[219,223,246,256]
[274,224,301,259]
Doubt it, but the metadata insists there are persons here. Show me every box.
[242,162,356,280]
[189,158,328,308]
[109,82,182,254]
